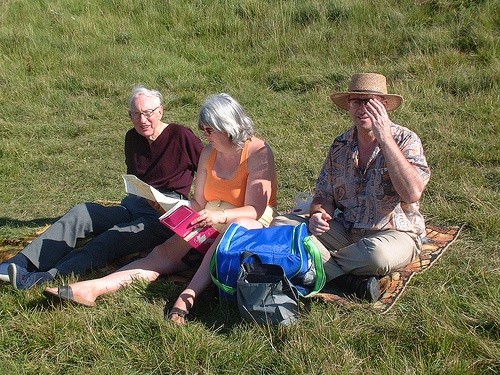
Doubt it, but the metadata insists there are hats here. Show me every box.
[330,72,403,112]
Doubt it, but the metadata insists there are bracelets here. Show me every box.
[309,211,322,219]
[221,209,228,224]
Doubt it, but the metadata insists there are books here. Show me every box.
[159,202,220,256]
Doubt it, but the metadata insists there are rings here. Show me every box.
[203,222,206,225]
[315,222,316,226]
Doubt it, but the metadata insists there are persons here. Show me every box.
[43,93,276,327]
[271,73,431,304]
[0,88,204,291]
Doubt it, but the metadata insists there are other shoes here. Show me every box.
[340,276,381,303]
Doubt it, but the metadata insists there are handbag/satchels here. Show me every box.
[210,222,310,305]
[237,250,311,327]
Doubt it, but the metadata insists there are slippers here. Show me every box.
[167,305,191,326]
[43,285,95,309]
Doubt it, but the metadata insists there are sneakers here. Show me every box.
[8,263,44,291]
[0,261,12,282]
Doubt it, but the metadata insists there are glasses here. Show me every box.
[348,98,370,108]
[199,124,214,134]
[129,106,160,120]
